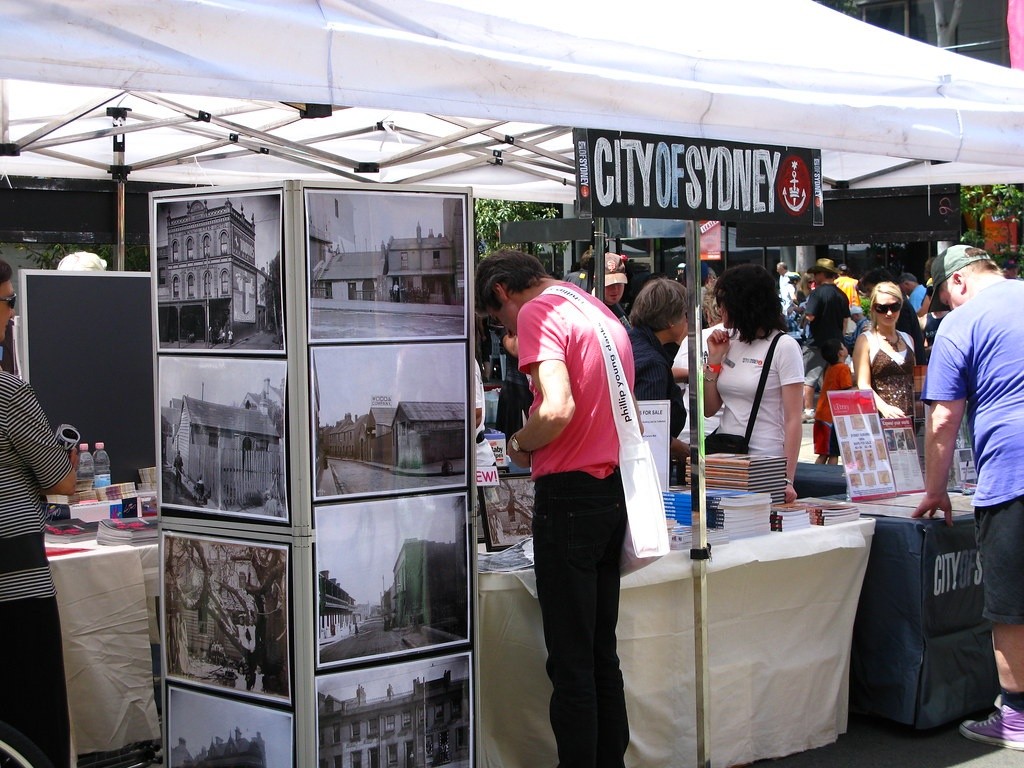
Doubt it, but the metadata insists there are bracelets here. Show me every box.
[703,376,718,382]
[706,362,722,373]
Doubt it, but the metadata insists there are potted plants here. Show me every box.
[961,182,1024,278]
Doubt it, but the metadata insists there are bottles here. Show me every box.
[92,443,111,487]
[75,443,94,492]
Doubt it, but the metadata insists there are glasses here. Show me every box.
[874,301,901,313]
[0,292,16,310]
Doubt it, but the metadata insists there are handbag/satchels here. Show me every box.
[705,434,749,455]
[618,439,670,576]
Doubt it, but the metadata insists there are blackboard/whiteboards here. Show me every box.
[18,268,163,488]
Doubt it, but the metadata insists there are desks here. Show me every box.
[475,517,876,768]
[804,490,999,734]
[40,514,160,594]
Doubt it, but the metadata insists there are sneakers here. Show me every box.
[959,695,1024,751]
[801,409,816,423]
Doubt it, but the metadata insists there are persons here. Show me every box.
[912,245,1024,751]
[211,612,266,693]
[321,614,391,639]
[476,250,645,768]
[262,490,278,516]
[208,326,233,349]
[474,252,952,501]
[389,280,430,303]
[173,450,183,494]
[0,260,80,768]
[193,473,204,505]
[324,457,328,470]
[409,742,449,766]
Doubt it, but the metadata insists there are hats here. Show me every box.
[605,252,627,287]
[928,245,990,313]
[807,258,842,274]
[676,263,686,269]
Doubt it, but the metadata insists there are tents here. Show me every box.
[0,0,1024,768]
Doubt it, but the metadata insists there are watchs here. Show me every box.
[511,435,534,454]
[784,478,793,485]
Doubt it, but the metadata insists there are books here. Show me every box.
[46,467,158,547]
[663,453,860,550]
[55,424,80,450]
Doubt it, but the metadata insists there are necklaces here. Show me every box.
[875,326,903,352]
